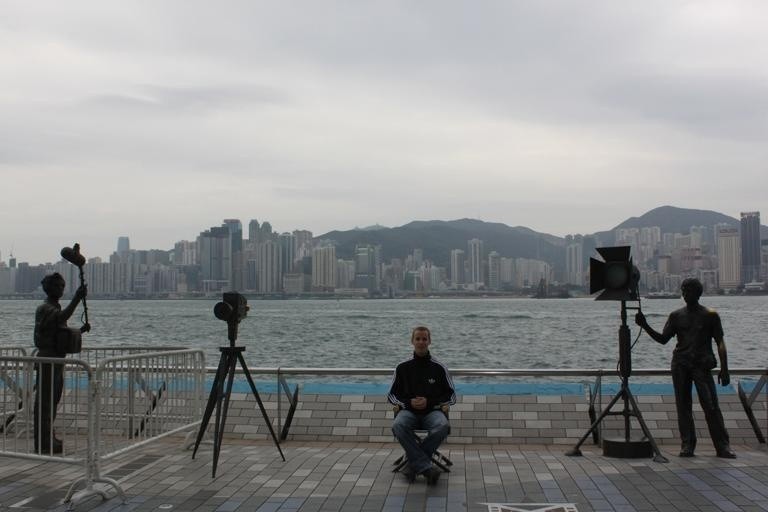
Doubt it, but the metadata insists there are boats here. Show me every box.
[644,291,679,299]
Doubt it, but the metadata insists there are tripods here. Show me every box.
[192,339,286,478]
[565,301,669,464]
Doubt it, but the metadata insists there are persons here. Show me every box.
[33,272,91,454]
[384,326,457,486]
[634,279,739,460]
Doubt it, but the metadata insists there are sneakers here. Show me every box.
[403,464,416,481]
[423,467,440,487]
[716,448,737,458]
[681,449,694,455]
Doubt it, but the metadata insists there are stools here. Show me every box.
[391,405,453,473]
